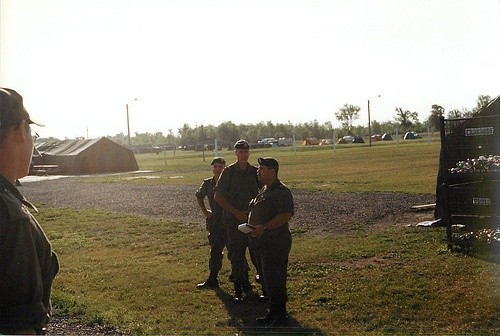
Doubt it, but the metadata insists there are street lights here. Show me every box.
[126,97,137,149]
[367,92,382,148]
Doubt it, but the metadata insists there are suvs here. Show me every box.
[258,136,278,146]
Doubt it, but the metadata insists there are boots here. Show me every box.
[197,269,219,288]
[234,281,244,303]
[255,272,288,329]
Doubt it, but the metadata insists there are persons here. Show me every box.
[0,87,60,335]
[195,157,253,294]
[238,157,294,322]
[214,139,265,302]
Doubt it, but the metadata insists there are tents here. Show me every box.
[303,131,422,146]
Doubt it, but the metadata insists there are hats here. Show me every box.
[234,140,250,149]
[258,157,279,167]
[211,157,226,165]
[0,87,45,127]
